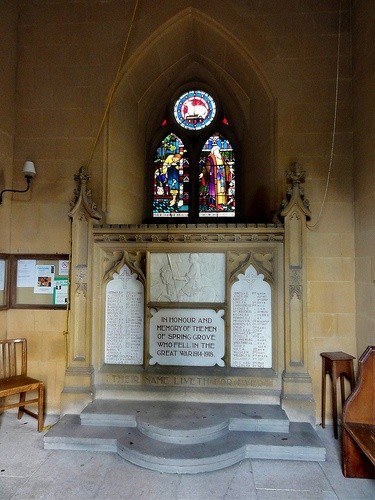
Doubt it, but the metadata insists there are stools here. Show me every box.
[320,350,356,441]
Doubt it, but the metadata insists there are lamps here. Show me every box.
[0,160,37,206]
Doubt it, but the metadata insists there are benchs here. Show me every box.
[338,345,375,480]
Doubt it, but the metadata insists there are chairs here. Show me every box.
[0,336,47,433]
